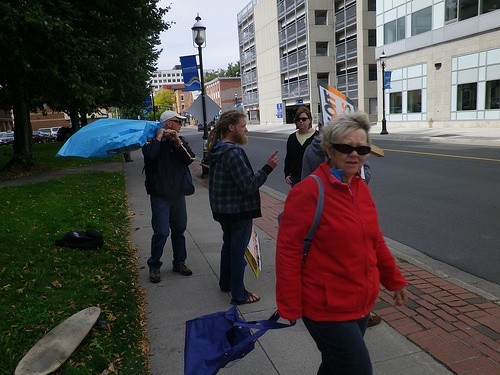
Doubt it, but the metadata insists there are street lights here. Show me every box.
[191,12,209,179]
[379,50,388,135]
[149,84,156,121]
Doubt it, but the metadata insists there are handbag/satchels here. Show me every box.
[184,306,297,375]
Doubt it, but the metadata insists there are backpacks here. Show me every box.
[55,230,104,250]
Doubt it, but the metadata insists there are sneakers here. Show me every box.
[150,269,161,283]
[173,264,193,276]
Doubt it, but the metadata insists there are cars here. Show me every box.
[198,121,211,131]
[0,131,14,145]
[32,131,45,143]
[51,127,61,138]
[38,128,56,143]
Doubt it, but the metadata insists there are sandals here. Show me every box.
[231,293,260,304]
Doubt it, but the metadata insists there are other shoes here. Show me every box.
[367,316,381,327]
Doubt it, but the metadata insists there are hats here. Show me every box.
[160,110,186,123]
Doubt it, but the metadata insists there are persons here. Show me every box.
[206,111,280,306]
[284,107,321,187]
[141,110,195,282]
[301,115,381,327]
[276,109,409,375]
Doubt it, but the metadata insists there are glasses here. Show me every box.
[329,142,371,155]
[170,119,183,125]
[297,117,310,122]
